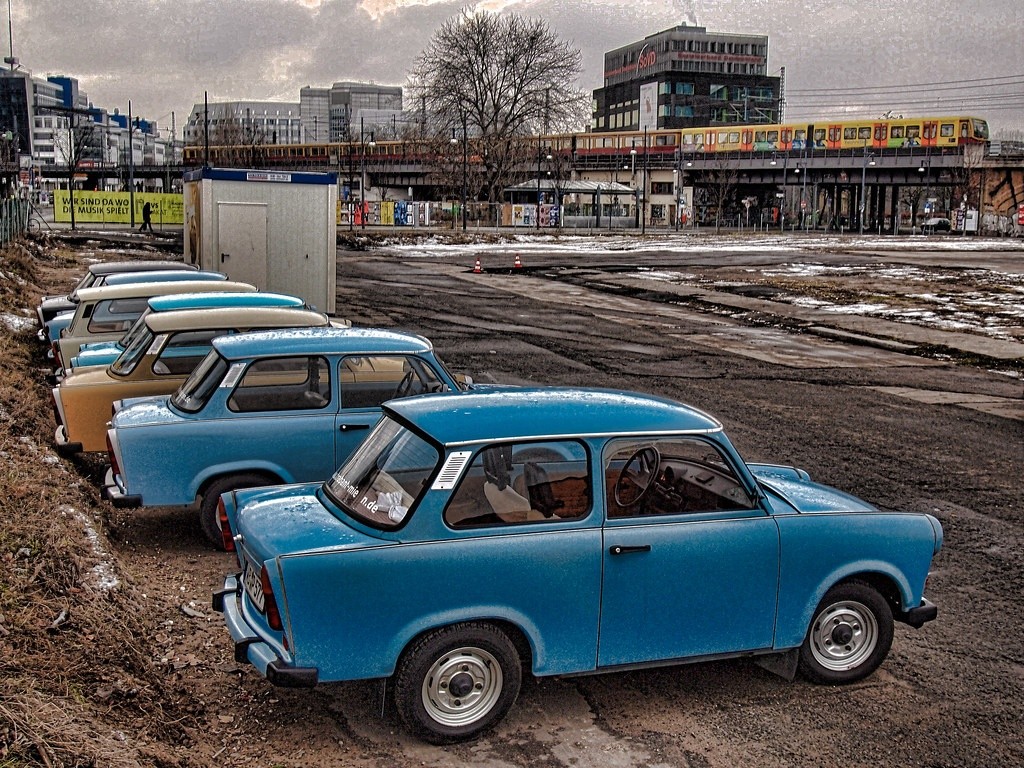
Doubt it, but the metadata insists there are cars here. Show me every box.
[48,308,473,456]
[921,217,952,233]
[36,260,351,388]
[98,326,583,552]
[213,384,947,745]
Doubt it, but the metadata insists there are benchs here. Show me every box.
[299,390,329,408]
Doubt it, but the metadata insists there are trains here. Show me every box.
[183,115,993,169]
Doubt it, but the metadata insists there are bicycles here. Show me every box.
[28,213,41,234]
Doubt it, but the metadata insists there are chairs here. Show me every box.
[522,462,565,520]
[479,447,530,521]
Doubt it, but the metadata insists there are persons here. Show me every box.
[139,203,153,231]
[122,181,129,192]
[136,180,143,193]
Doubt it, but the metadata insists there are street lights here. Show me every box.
[359,115,376,230]
[514,252,523,268]
[536,133,552,231]
[473,257,485,276]
[126,99,145,229]
[919,147,934,235]
[795,151,805,232]
[858,138,878,239]
[770,152,786,232]
[450,126,459,231]
[608,158,629,229]
[675,157,692,232]
